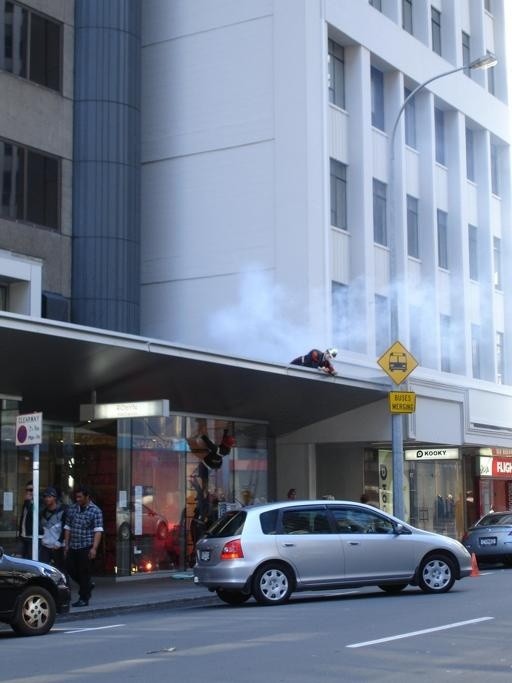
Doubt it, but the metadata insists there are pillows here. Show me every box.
[222,434,235,448]
[360,494,369,501]
[288,488,297,495]
[327,348,338,358]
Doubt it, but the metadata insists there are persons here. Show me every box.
[19,481,103,606]
[290,347,338,375]
[288,488,297,501]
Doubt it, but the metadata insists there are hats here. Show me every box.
[190,499,474,607]
[460,508,512,572]
[1,547,72,637]
[116,499,172,543]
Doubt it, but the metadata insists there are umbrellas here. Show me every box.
[71,582,96,607]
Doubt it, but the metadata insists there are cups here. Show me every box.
[25,488,33,492]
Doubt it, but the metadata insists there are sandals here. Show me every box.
[387,351,409,372]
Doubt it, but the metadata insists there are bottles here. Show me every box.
[42,487,58,497]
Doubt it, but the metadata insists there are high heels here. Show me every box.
[469,552,481,577]
